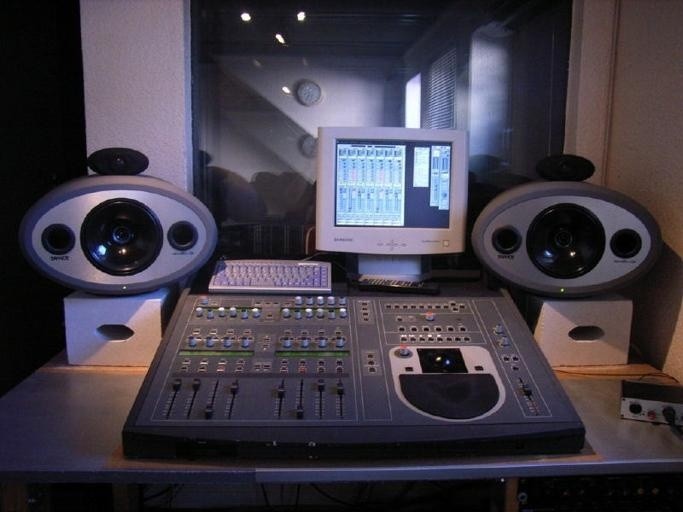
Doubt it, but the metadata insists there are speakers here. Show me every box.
[20,146,217,297]
[471,155,662,294]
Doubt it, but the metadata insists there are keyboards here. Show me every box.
[209,257,332,294]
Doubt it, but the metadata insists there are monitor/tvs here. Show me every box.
[314,125,469,279]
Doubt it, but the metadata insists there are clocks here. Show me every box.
[297,79,323,107]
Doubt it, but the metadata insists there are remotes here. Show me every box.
[349,274,441,295]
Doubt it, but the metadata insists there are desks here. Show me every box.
[1,347,681,511]
